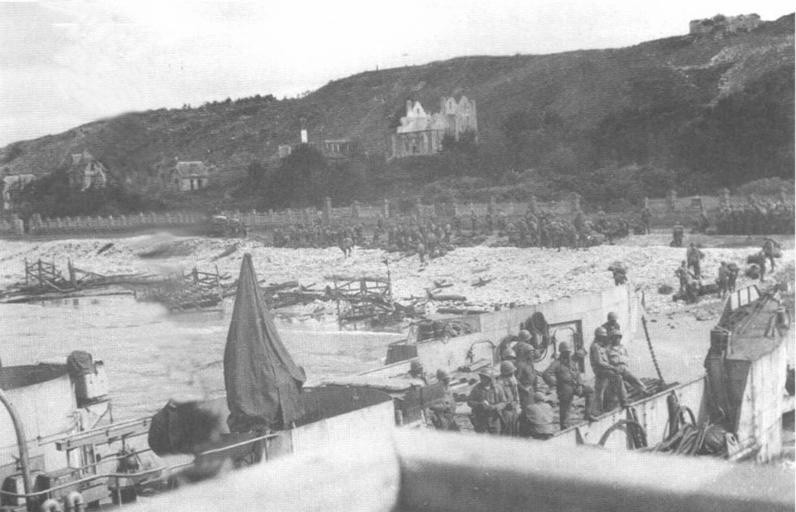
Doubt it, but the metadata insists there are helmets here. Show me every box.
[559,341,571,352]
[595,312,622,338]
[478,330,534,380]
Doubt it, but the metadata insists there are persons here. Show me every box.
[268,208,792,302]
[404,311,649,440]
